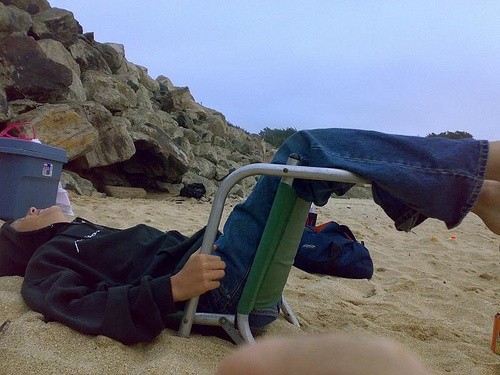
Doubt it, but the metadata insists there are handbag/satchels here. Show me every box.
[295,219,373,281]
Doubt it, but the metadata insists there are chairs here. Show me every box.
[172,154,380,347]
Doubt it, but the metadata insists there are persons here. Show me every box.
[0,128,500,347]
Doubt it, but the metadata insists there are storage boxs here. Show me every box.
[0,137,70,223]
